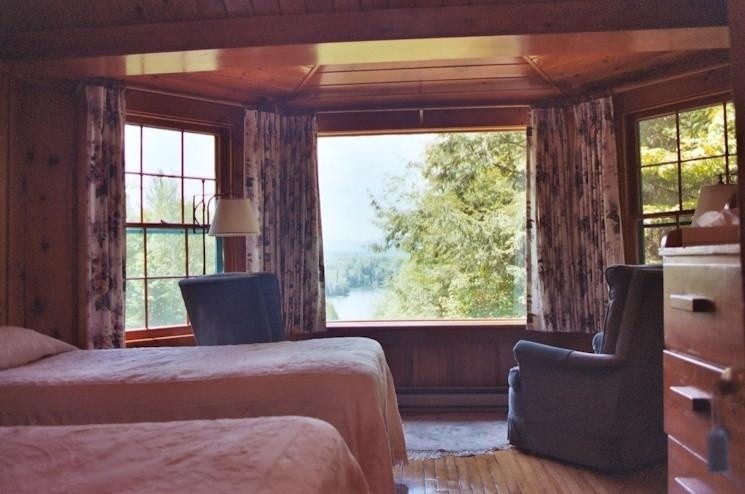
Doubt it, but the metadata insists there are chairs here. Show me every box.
[178,272,285,346]
[508,264,663,477]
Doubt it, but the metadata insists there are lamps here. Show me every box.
[201,193,261,275]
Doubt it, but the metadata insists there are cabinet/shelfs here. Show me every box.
[657,244,745,493]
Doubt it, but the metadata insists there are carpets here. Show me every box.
[398,405,510,462]
